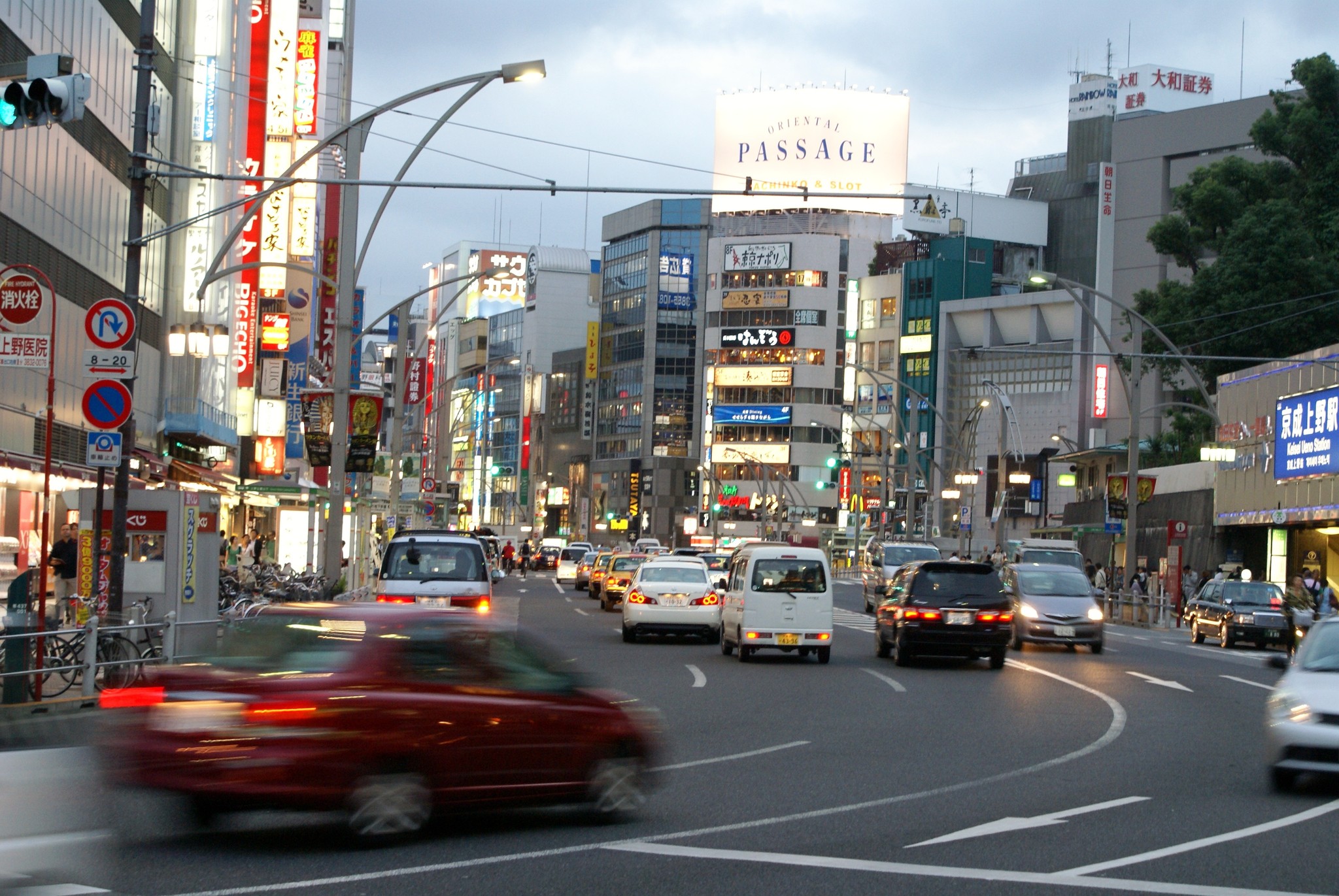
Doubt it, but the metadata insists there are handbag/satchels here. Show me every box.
[220,539,227,556]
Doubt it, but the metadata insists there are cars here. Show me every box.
[997,561,1104,654]
[91,599,667,849]
[1182,577,1296,651]
[1262,612,1339,798]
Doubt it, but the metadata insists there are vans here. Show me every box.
[373,528,836,666]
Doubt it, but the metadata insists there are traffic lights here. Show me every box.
[490,465,514,475]
[0,72,92,131]
[974,466,984,476]
[699,512,710,527]
[816,481,836,490]
[714,504,730,517]
[607,513,621,523]
[827,458,852,468]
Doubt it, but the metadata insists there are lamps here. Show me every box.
[1049,435,1082,454]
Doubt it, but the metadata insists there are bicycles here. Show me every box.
[0,591,179,699]
[218,560,340,625]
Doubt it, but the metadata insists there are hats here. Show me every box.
[507,541,511,544]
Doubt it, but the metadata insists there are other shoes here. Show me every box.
[66,618,71,624]
[58,619,64,628]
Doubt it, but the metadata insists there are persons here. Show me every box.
[1198,569,1215,589]
[1085,559,1123,608]
[341,541,349,568]
[220,528,275,572]
[1228,565,1266,581]
[949,552,971,561]
[1182,566,1198,601]
[519,539,532,575]
[1280,574,1315,659]
[781,565,816,582]
[1301,567,1334,620]
[131,534,165,561]
[980,544,1008,579]
[501,540,515,573]
[1215,568,1226,578]
[1129,566,1152,602]
[48,522,78,627]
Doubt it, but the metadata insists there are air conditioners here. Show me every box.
[1077,490,1084,502]
[1087,486,1101,500]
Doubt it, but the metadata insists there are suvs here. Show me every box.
[861,539,943,615]
[873,559,1013,671]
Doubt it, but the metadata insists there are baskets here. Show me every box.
[1293,612,1312,626]
[96,611,130,633]
[241,581,255,590]
[147,617,167,638]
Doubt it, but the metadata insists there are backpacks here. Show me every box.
[522,544,530,555]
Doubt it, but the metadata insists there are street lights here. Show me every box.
[317,264,511,547]
[475,478,533,536]
[1026,267,1237,623]
[696,465,736,553]
[169,59,549,601]
[809,363,1032,567]
[725,446,817,543]
[547,470,604,542]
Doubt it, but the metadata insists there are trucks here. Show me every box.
[1004,538,1087,576]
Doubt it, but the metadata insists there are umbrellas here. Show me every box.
[235,475,371,506]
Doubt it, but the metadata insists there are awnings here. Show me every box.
[135,449,250,500]
